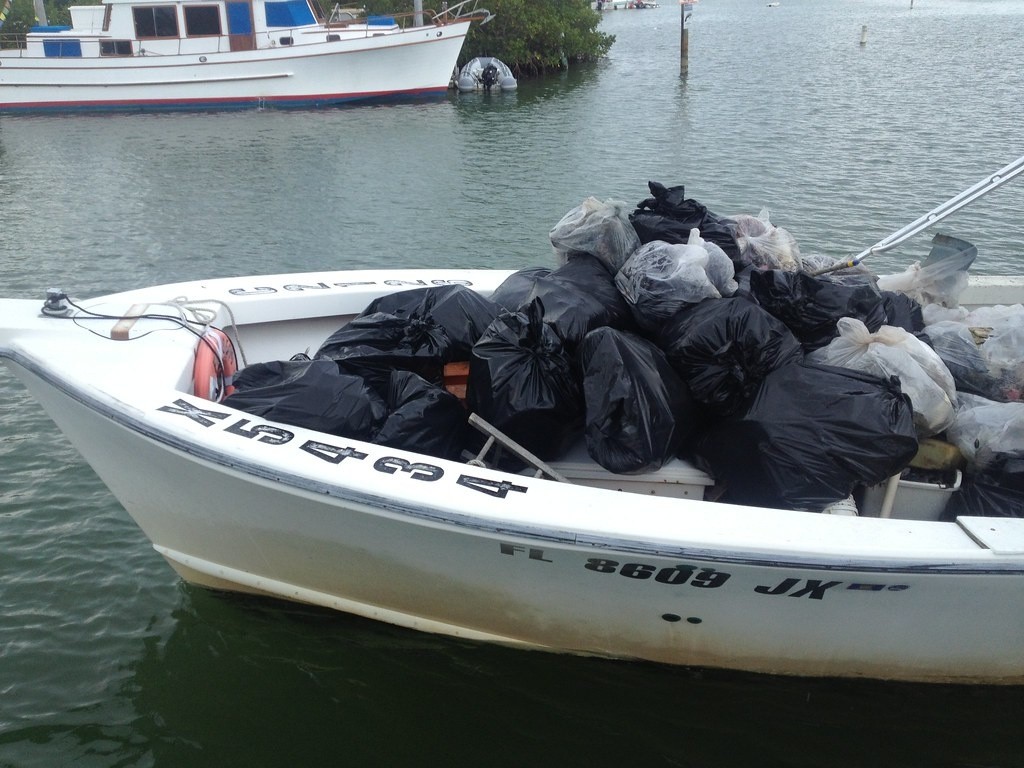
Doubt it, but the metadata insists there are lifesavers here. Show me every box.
[194,331,238,402]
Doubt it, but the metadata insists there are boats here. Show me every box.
[1,252,1024,691]
[0,0,476,115]
